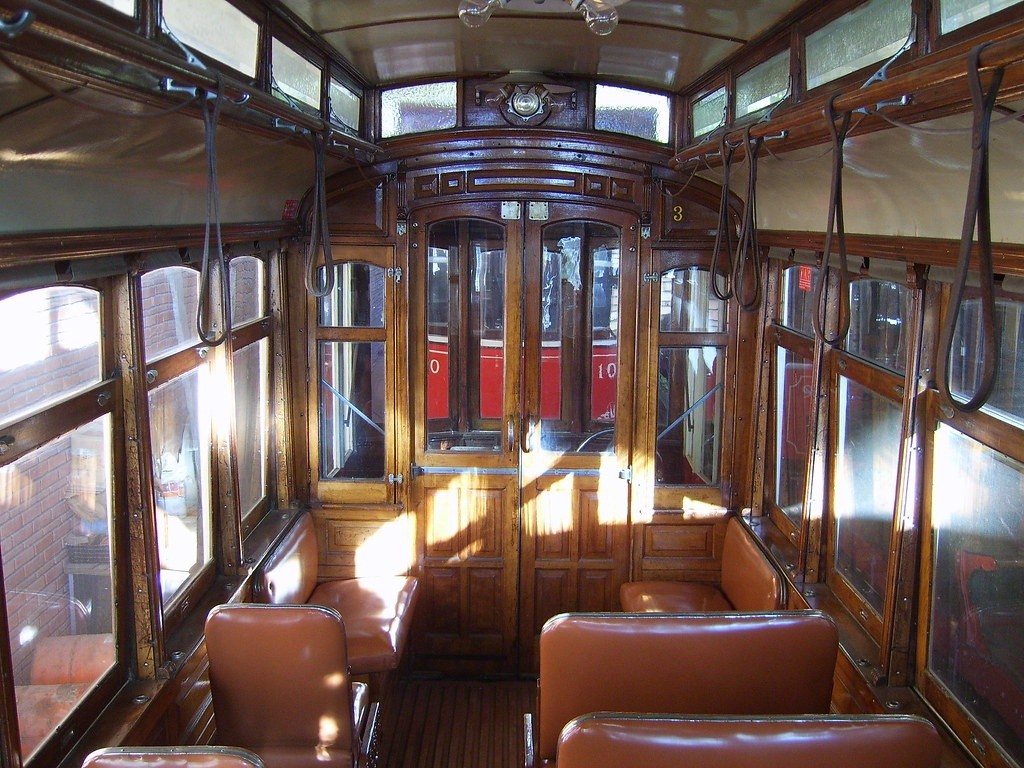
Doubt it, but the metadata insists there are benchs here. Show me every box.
[252,502,946,768]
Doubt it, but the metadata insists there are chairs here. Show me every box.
[79,602,384,768]
